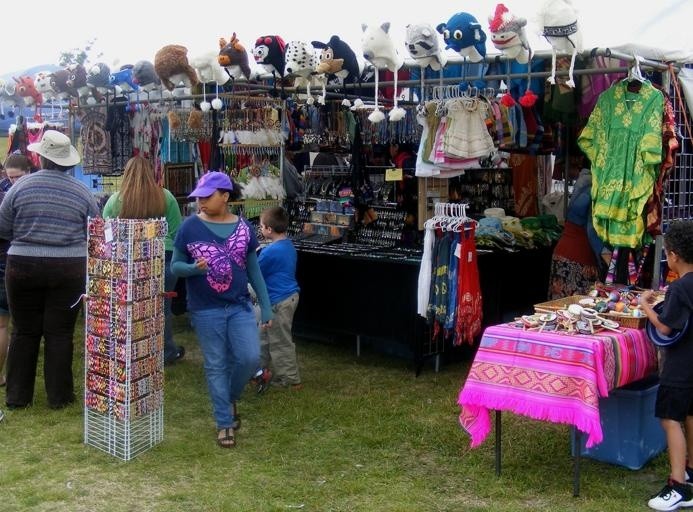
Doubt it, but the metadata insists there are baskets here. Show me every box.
[534,294,648,330]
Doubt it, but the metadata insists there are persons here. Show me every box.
[639,220,691,511]
[366,145,391,167]
[0,190,9,389]
[386,142,416,171]
[101,154,185,365]
[246,206,304,391]
[0,150,40,193]
[0,130,100,408]
[233,151,283,221]
[270,151,305,200]
[169,171,274,448]
[546,169,612,301]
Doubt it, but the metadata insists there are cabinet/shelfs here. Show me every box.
[452,169,514,219]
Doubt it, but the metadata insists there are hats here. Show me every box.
[475,207,564,252]
[27,130,81,167]
[187,172,233,198]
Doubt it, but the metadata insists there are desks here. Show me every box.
[442,228,563,373]
[243,231,445,373]
[454,283,672,500]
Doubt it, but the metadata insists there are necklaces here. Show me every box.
[309,178,395,207]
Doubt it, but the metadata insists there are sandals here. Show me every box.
[231,401,241,430]
[216,426,236,448]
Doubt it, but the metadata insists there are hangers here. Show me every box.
[422,200,478,233]
[624,62,644,82]
[618,57,646,104]
[544,178,574,198]
[416,83,514,114]
[7,112,67,136]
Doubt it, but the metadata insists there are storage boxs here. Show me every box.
[569,377,669,473]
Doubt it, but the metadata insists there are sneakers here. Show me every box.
[165,345,185,366]
[648,468,693,511]
[256,369,272,393]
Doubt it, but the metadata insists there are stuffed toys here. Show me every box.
[1,1,584,122]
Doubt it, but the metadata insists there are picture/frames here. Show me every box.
[164,162,196,204]
